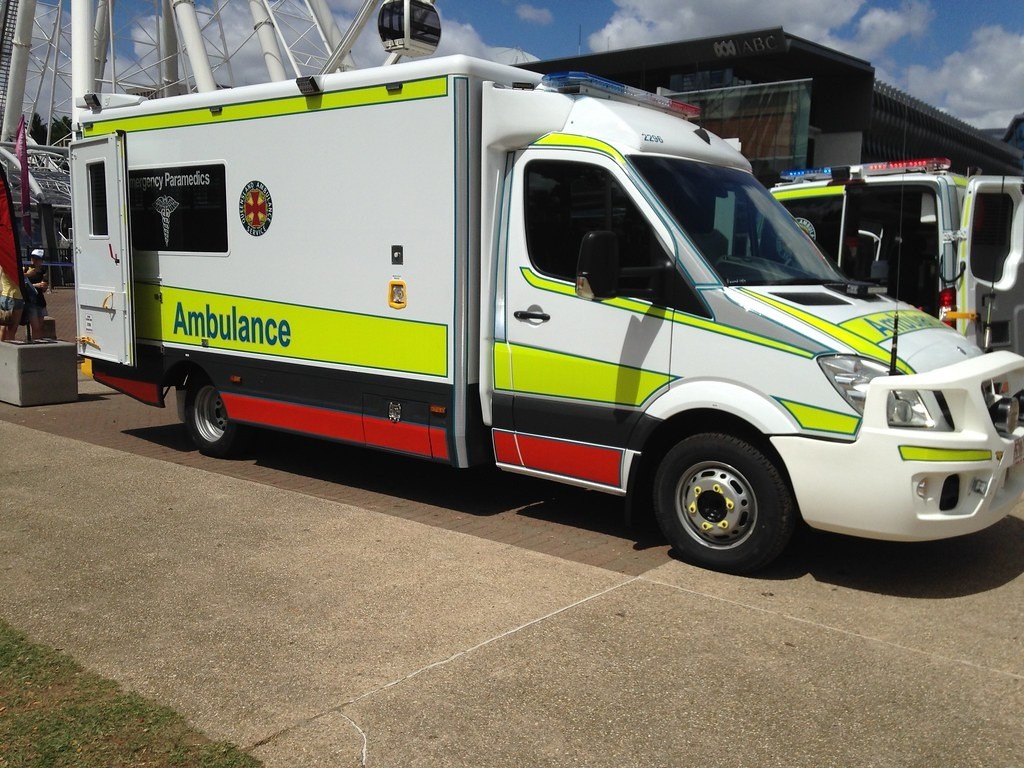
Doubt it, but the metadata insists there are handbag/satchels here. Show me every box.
[18,304,31,326]
[0,305,16,326]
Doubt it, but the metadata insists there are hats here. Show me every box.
[30,249,45,259]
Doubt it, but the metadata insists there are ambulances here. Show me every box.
[754,152,1022,359]
[66,52,1024,576]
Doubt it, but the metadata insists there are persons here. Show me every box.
[0,262,24,343]
[22,249,50,340]
